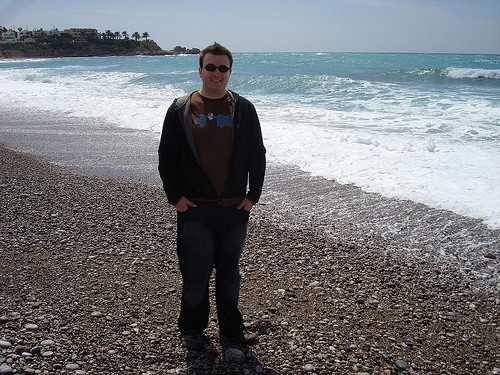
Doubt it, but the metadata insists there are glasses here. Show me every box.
[202,63,230,73]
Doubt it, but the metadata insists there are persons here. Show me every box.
[158,43,266,349]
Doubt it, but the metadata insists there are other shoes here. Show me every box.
[181,334,203,349]
[223,344,248,358]
